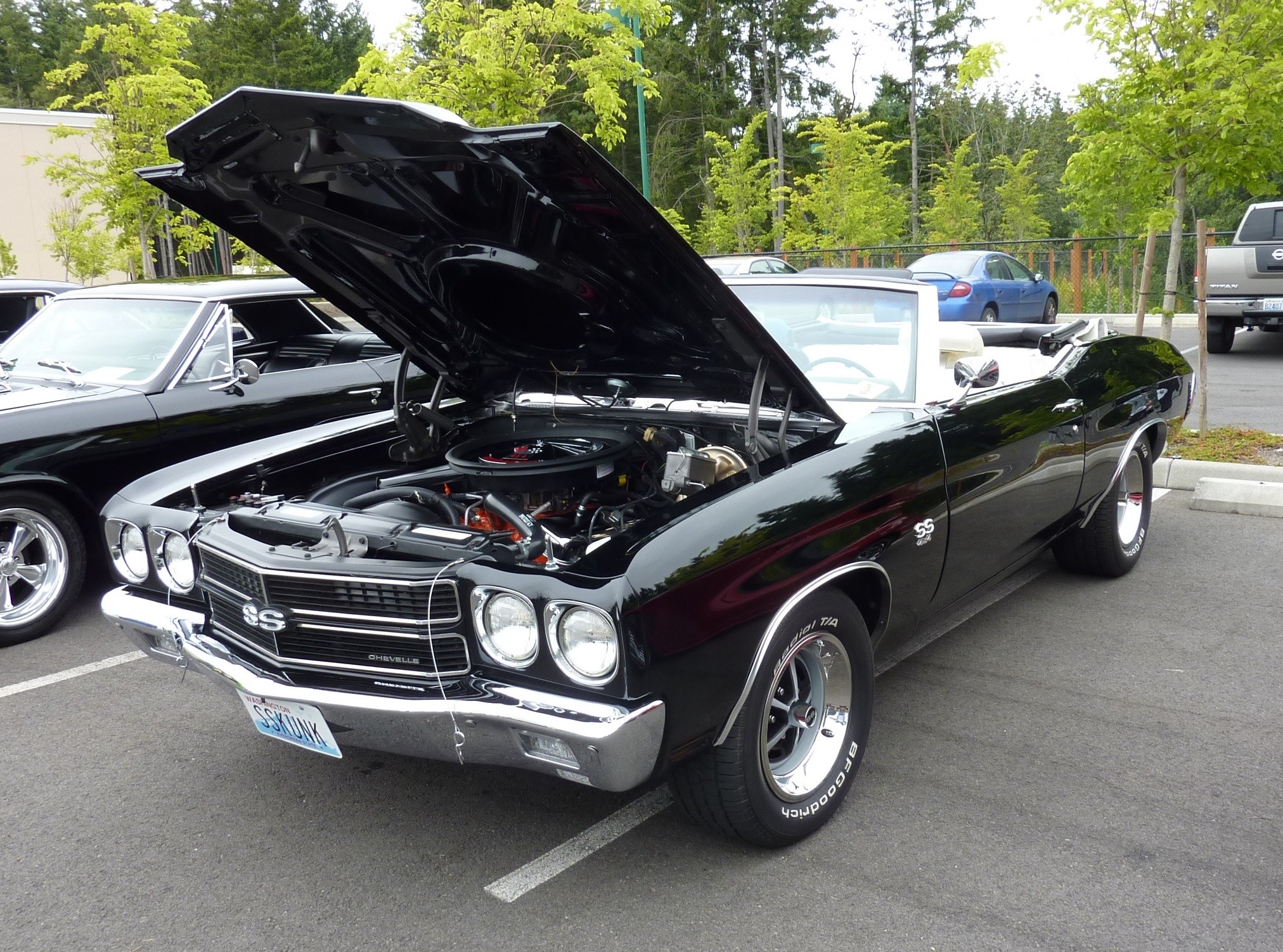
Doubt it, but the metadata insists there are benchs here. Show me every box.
[250,332,397,377]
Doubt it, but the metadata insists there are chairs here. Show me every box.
[935,323,985,394]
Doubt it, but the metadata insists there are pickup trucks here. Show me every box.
[1193,201,1283,353]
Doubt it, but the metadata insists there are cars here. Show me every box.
[0,85,1196,846]
[907,249,1060,323]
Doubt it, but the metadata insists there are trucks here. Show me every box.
[1,109,132,289]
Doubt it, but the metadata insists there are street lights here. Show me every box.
[809,141,828,236]
[600,7,650,205]
